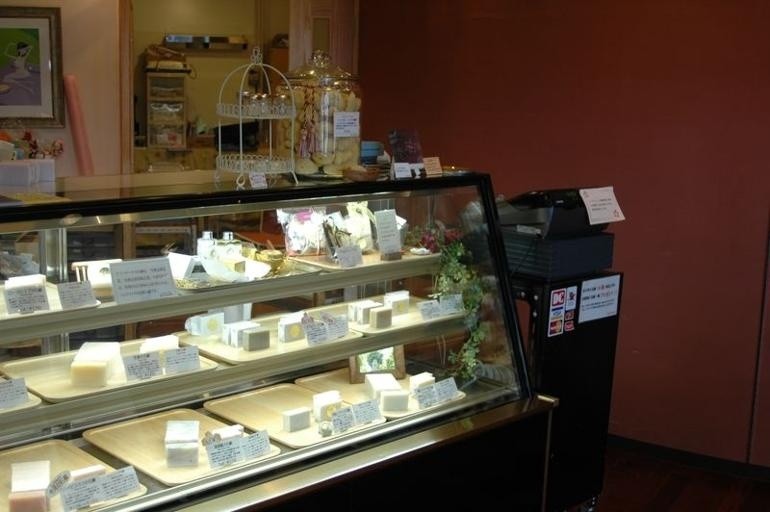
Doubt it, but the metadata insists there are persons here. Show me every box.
[1,42,37,98]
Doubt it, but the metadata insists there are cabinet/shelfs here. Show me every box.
[0,160,561,512]
[493,265,626,510]
[143,71,190,153]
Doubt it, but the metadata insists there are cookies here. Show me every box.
[276,86,361,177]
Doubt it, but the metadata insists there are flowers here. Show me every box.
[404,219,486,384]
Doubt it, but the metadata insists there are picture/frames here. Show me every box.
[0,5,66,133]
